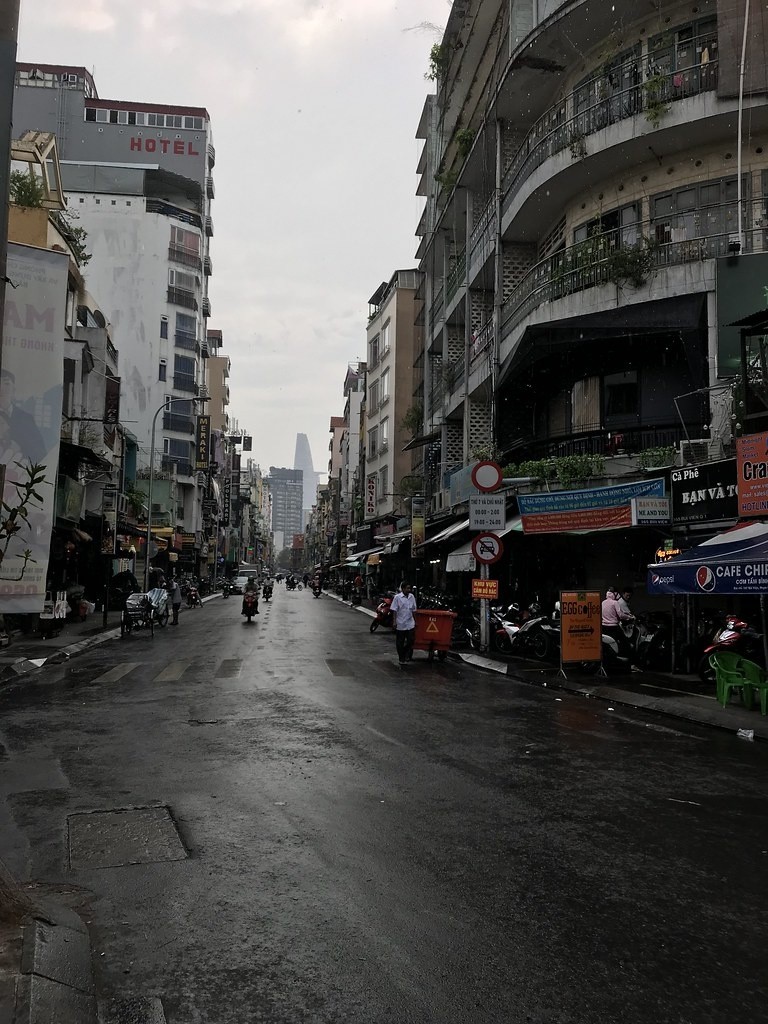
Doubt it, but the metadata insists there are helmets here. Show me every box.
[248,576,254,580]
[315,576,318,579]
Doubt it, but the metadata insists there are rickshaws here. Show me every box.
[121,588,170,638]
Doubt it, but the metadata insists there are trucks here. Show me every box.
[238,565,263,587]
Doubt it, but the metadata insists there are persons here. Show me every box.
[185,576,204,608]
[241,575,260,614]
[313,576,322,594]
[355,573,363,597]
[389,581,417,666]
[167,579,181,625]
[275,572,297,591]
[262,576,274,598]
[596,583,647,672]
[303,574,309,588]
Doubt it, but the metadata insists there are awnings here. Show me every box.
[345,547,384,564]
[413,519,469,548]
[646,521,768,596]
[446,516,521,572]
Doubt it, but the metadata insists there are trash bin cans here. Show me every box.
[414,608,457,662]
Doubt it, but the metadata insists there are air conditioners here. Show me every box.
[681,439,713,465]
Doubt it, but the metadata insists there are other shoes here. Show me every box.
[201,606,204,608]
[256,610,260,614]
[241,609,244,614]
[631,665,643,672]
[262,595,265,598]
[171,621,178,625]
[405,658,409,662]
[398,659,407,664]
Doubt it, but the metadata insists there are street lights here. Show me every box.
[143,395,213,593]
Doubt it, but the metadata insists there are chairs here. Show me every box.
[740,658,768,716]
[714,651,753,708]
[709,654,746,706]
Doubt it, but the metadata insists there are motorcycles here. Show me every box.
[286,580,303,591]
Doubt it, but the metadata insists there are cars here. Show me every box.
[233,576,248,591]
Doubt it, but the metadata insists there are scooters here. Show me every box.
[241,591,260,623]
[310,583,323,598]
[189,587,199,609]
[603,602,764,688]
[370,584,562,659]
[263,584,273,602]
[199,575,232,599]
